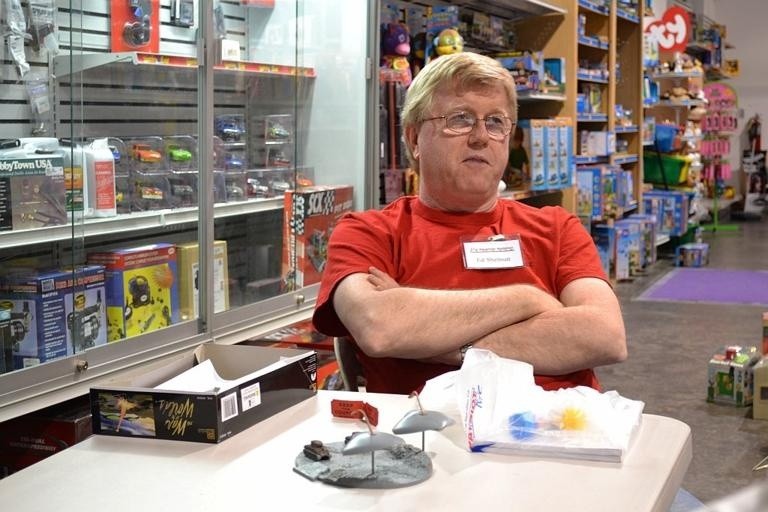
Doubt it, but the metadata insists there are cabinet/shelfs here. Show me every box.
[378,0,743,270]
[2,0,367,422]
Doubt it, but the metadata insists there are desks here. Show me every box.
[0,385,696,509]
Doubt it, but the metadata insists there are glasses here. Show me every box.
[417,111,517,137]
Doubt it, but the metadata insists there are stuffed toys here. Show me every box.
[434,29,466,58]
[660,45,713,188]
[379,21,414,90]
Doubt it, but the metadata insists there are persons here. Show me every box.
[307,49,709,512]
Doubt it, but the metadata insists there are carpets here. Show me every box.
[628,265,767,308]
